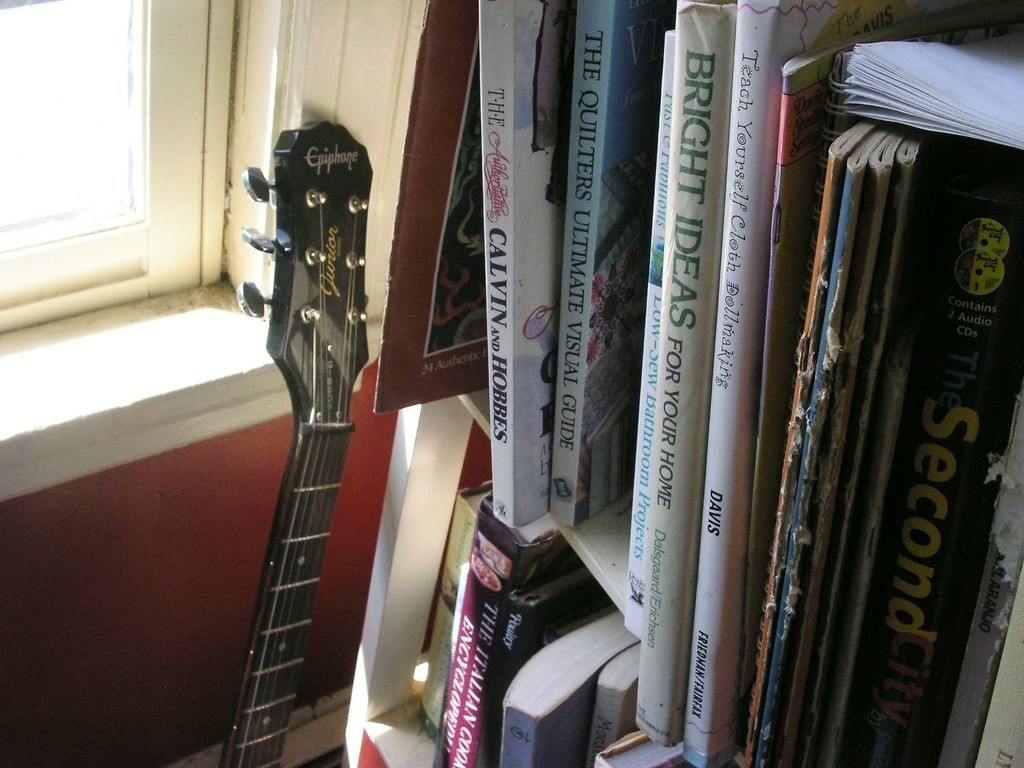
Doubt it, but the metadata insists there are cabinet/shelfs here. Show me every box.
[344,389,632,768]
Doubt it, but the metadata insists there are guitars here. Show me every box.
[215,116,377,768]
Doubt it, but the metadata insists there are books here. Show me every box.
[373,0,1024,768]
